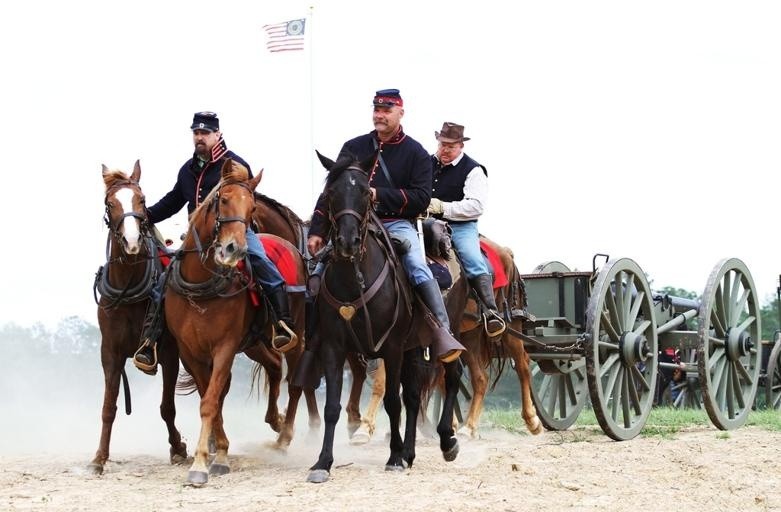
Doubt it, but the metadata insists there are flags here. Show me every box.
[260,17,308,58]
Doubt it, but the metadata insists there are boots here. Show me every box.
[416,278,461,363]
[267,282,295,348]
[136,301,166,375]
[470,274,502,343]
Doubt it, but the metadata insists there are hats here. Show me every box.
[191,111,219,132]
[435,122,470,143]
[373,89,403,108]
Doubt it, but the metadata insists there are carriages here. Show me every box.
[85,147,781,488]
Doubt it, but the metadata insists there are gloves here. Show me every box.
[424,198,444,213]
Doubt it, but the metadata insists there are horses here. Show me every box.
[344,233,540,443]
[247,187,367,451]
[306,148,469,493]
[163,158,310,487]
[87,158,188,481]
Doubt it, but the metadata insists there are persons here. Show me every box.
[423,121,505,336]
[133,111,295,376]
[305,87,455,339]
